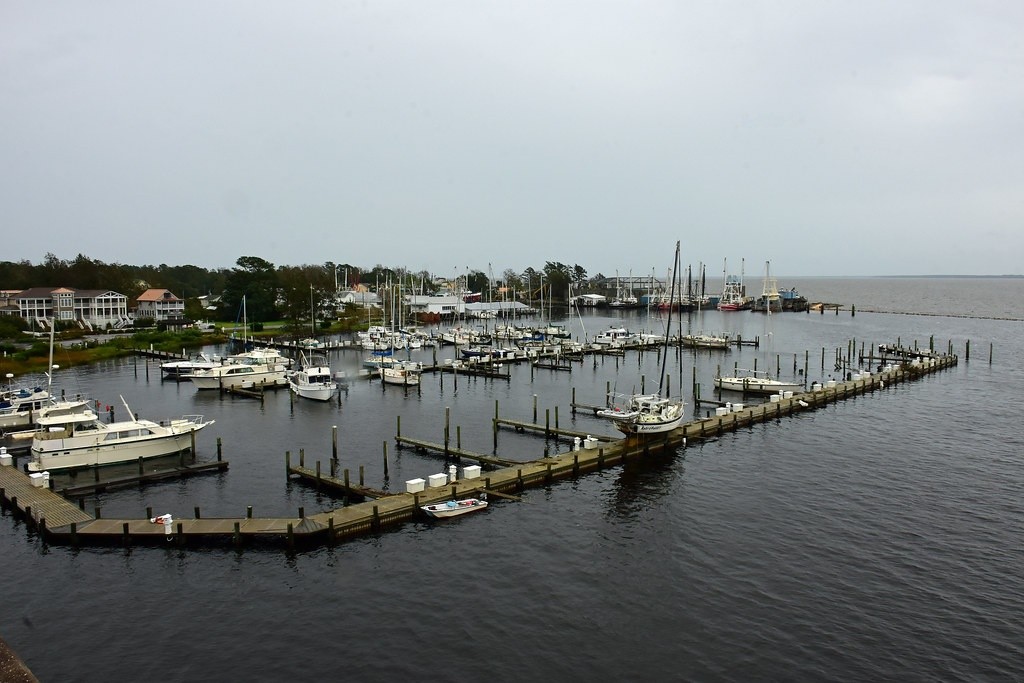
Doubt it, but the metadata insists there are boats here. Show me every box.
[715,372,805,395]
[0,254,818,472]
[421,500,490,518]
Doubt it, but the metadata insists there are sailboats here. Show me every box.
[594,238,688,434]
